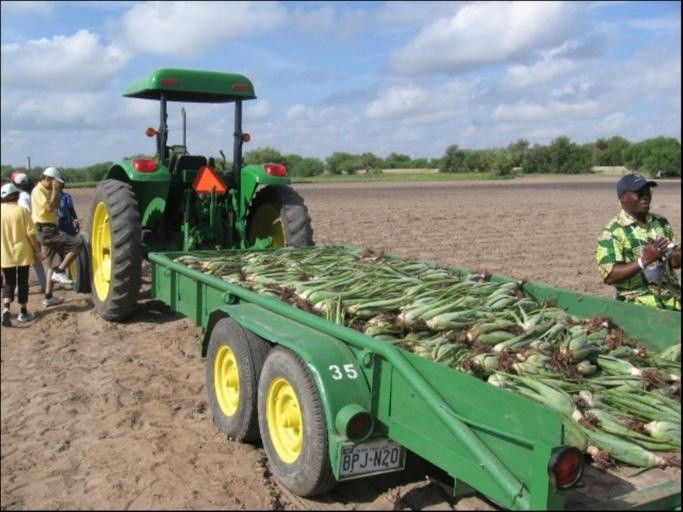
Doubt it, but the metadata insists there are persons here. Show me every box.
[31,167,83,306]
[10,171,47,292]
[57,182,80,279]
[596,173,681,310]
[1,183,45,325]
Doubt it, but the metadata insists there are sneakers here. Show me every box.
[51,271,73,284]
[2,308,35,326]
[44,296,63,305]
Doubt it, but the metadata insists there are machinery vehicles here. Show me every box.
[69,69,314,324]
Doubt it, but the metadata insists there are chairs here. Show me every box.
[174,155,207,177]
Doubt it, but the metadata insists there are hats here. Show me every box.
[617,174,657,194]
[1,167,64,198]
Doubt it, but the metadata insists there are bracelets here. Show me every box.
[638,256,645,270]
